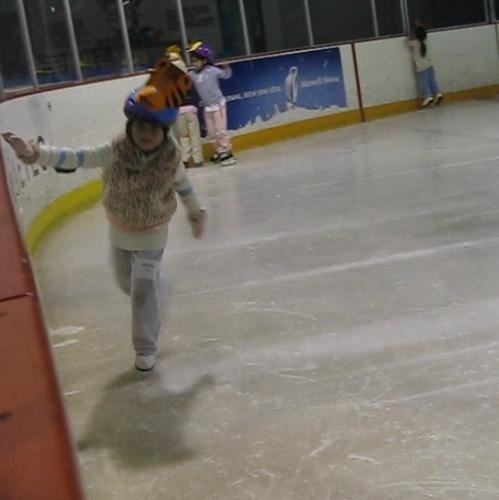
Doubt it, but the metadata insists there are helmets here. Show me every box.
[192,44,215,62]
[120,83,178,125]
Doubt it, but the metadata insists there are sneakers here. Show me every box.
[134,354,156,371]
[209,152,234,165]
[421,94,443,108]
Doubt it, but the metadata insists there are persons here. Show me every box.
[403,24,444,110]
[180,39,239,166]
[159,48,208,167]
[2,81,209,373]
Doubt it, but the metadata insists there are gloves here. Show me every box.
[186,207,209,241]
[0,129,34,158]
